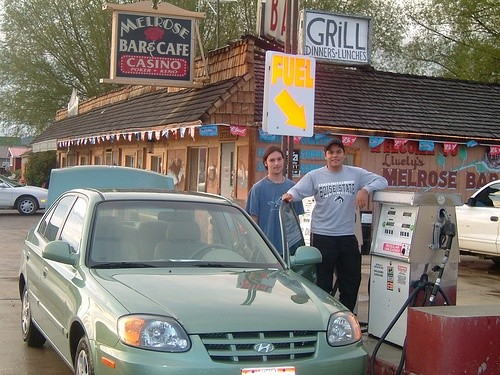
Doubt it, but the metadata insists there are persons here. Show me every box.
[245,144,306,258]
[282,137,389,313]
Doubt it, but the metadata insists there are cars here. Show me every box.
[455,179,500,262]
[0,174,49,216]
[18,165,367,375]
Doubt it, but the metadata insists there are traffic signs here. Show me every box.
[262,50,316,138]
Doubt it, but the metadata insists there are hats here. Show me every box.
[324,138,344,152]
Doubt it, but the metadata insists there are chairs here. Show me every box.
[136,221,169,261]
[91,215,123,263]
[153,221,212,259]
